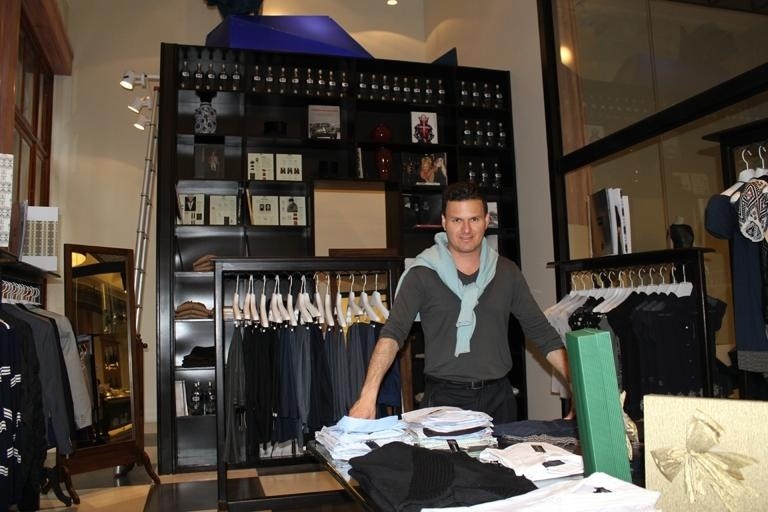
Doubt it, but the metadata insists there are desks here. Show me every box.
[306,421,643,511]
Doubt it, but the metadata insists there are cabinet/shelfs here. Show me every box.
[155,38,531,480]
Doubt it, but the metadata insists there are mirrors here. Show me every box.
[43,241,163,503]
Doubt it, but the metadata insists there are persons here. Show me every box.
[413,114,434,141]
[403,194,432,226]
[184,196,196,211]
[407,154,447,185]
[286,197,298,214]
[206,150,219,172]
[341,179,578,424]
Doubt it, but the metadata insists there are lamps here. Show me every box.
[120,70,160,131]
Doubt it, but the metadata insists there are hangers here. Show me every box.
[701,146,768,207]
[232,268,390,334]
[0,277,70,330]
[540,263,727,328]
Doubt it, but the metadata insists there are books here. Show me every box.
[586,186,628,258]
[621,195,632,255]
